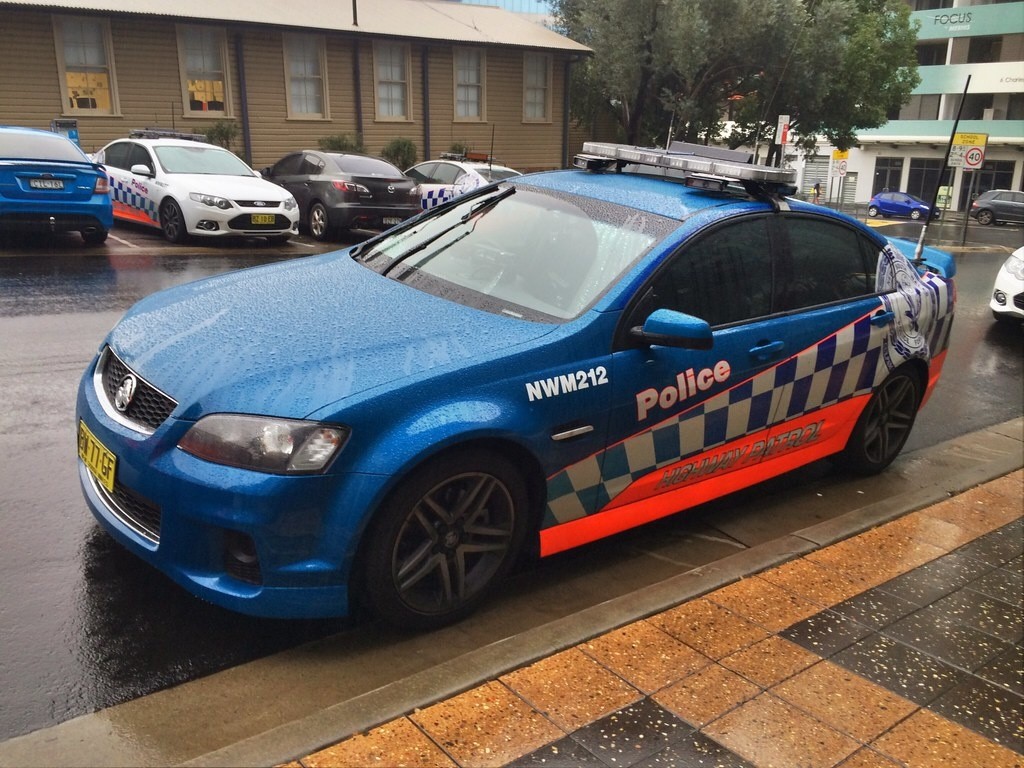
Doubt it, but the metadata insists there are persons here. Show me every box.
[811,184,819,204]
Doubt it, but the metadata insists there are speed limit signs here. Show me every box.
[961,146,986,169]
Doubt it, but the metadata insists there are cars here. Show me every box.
[868,191,941,221]
[400,149,535,213]
[258,149,423,242]
[83,126,302,243]
[0,124,114,244]
[989,245,1024,322]
[73,137,961,629]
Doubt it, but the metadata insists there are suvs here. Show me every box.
[969,189,1024,227]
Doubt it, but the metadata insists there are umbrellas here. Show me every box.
[807,178,822,185]
